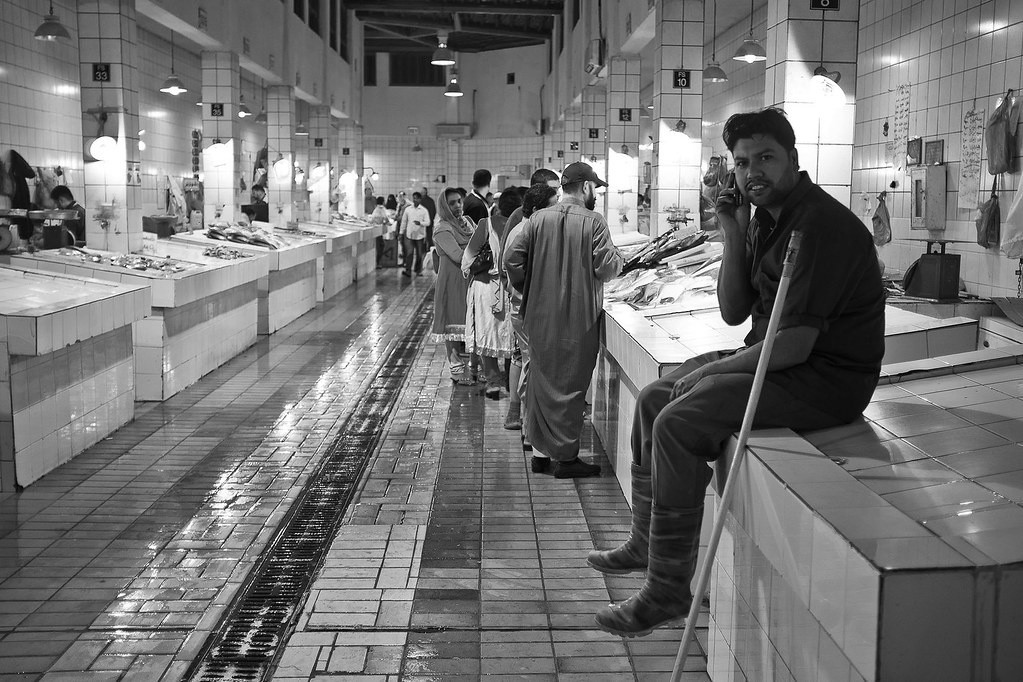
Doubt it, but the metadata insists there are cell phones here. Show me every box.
[734,177,743,206]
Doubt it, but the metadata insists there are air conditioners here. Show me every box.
[436,125,473,139]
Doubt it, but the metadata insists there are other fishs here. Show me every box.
[62,247,185,274]
[202,219,288,250]
[603,226,711,308]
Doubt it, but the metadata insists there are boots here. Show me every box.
[594,503,705,638]
[587,462,651,574]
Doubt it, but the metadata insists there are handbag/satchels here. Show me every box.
[468,218,494,274]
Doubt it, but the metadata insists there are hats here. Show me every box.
[561,162,609,187]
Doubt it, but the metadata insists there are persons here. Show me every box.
[29,185,85,246]
[502,161,624,478]
[432,169,529,401]
[421,187,436,261]
[497,168,562,429]
[586,104,886,638]
[399,191,431,277]
[365,188,412,267]
[252,184,268,204]
[242,209,256,225]
[503,183,558,452]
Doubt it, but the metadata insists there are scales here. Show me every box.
[29,210,81,249]
[896,237,980,302]
[0,208,28,255]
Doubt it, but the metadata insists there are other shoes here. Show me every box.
[450,376,475,385]
[478,369,487,383]
[486,385,500,399]
[415,270,424,276]
[402,270,411,277]
[504,402,522,429]
[550,459,601,479]
[531,455,550,473]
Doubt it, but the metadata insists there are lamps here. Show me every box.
[430,28,457,66]
[208,52,228,160]
[90,1,118,160]
[237,67,252,116]
[810,68,846,111]
[35,0,72,41]
[362,166,379,181]
[159,28,188,95]
[273,85,289,177]
[587,0,768,174]
[255,77,267,122]
[294,99,308,136]
[444,66,463,97]
